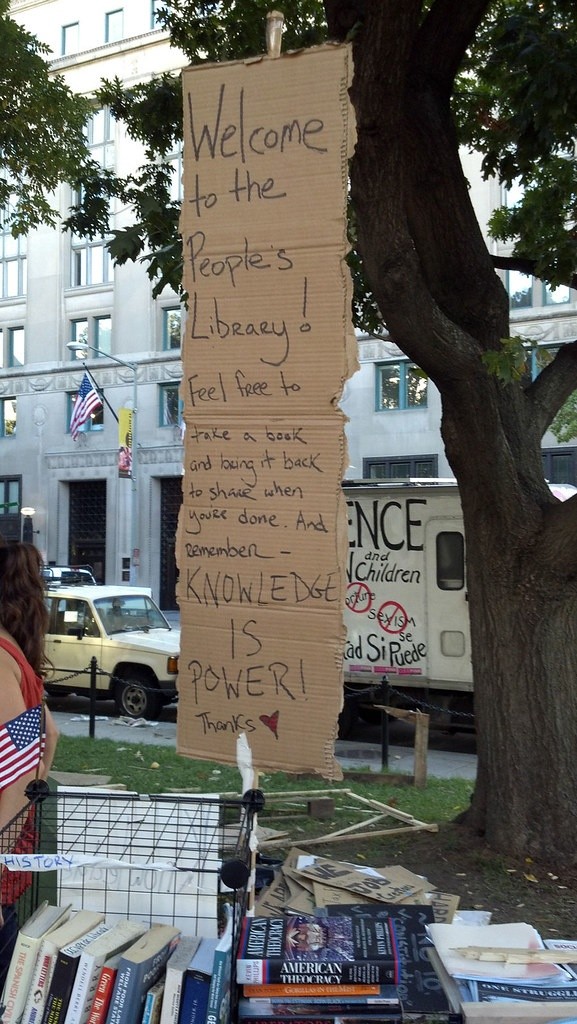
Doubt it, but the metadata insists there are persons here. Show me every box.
[120,447,131,471]
[0,535,59,984]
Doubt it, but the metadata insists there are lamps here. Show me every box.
[21,505,40,543]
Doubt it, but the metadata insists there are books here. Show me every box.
[0,900,577,1024]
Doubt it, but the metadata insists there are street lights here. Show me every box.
[66,341,141,587]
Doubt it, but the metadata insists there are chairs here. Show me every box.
[88,608,111,636]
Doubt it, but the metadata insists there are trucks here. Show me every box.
[338,477,577,740]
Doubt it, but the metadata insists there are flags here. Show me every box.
[70,373,102,441]
[0,704,46,792]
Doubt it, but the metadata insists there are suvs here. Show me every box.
[39,565,181,720]
